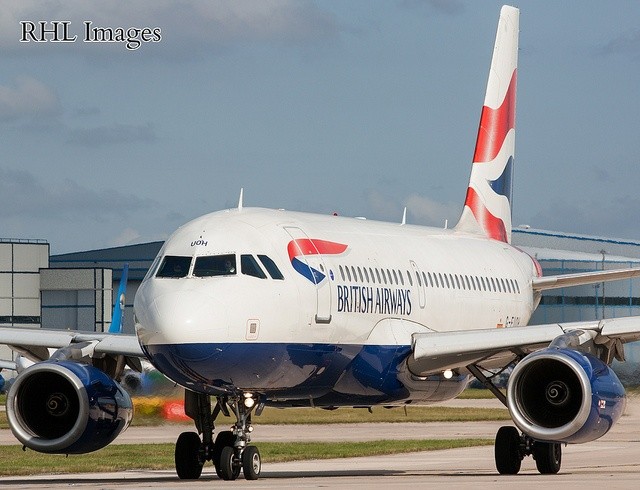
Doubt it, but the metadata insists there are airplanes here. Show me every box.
[0,5,640,481]
